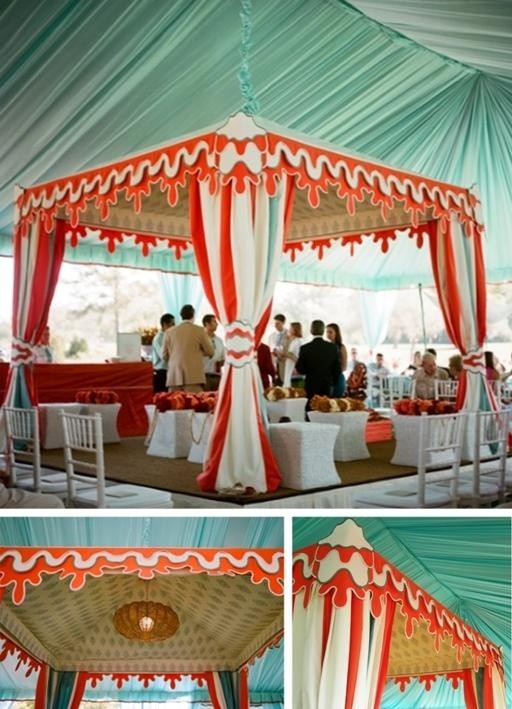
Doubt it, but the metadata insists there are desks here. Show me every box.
[0,360,156,438]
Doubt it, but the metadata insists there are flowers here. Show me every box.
[139,327,158,346]
[75,382,368,415]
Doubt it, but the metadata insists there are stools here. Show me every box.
[36,398,372,493]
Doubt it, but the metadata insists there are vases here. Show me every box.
[140,345,155,361]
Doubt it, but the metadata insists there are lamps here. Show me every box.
[111,579,181,644]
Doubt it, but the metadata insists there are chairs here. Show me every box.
[1,406,97,508]
[57,407,177,507]
[350,372,512,508]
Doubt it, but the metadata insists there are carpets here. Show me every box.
[2,433,511,507]
